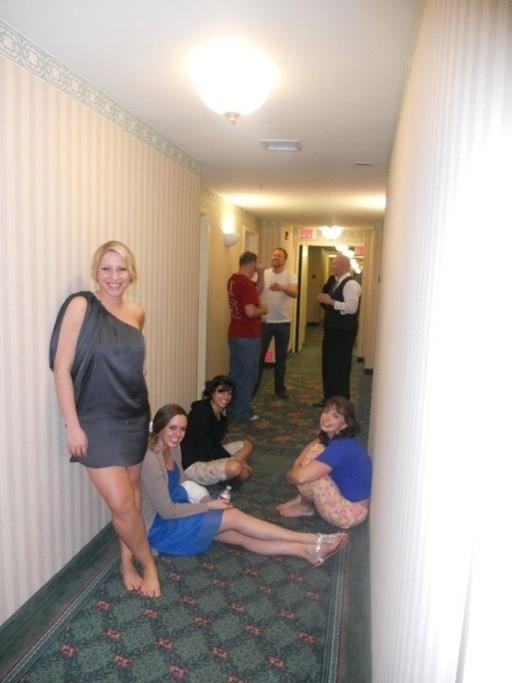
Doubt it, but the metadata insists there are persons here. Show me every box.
[48,241,163,599]
[140,404,349,568]
[255,246,300,397]
[275,397,370,531]
[179,374,254,486]
[313,256,363,408]
[223,250,268,421]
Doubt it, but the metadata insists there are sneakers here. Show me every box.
[275,385,289,399]
[251,415,260,421]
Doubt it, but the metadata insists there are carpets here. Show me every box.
[0,372,361,682]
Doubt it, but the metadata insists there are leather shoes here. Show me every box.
[312,399,326,407]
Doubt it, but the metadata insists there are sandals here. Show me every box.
[311,532,348,568]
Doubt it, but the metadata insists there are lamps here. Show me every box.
[224,233,240,246]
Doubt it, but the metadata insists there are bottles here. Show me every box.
[218,485,232,504]
